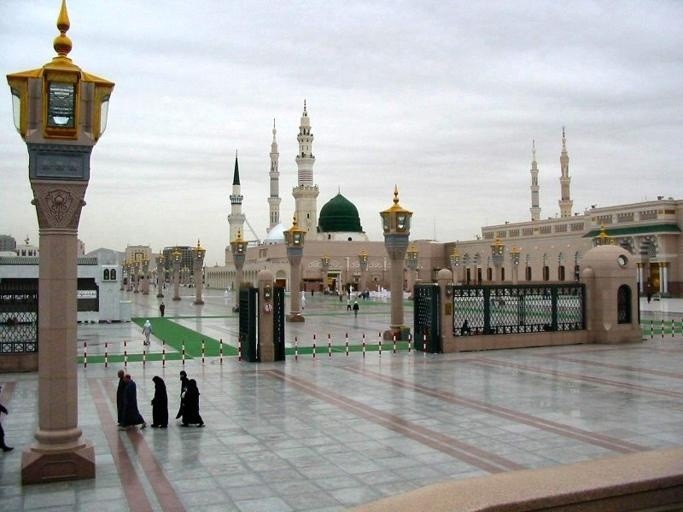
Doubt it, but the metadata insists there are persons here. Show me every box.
[311,288,360,318]
[118,374,147,430]
[116,369,125,427]
[180,378,204,427]
[150,375,168,428]
[159,301,165,316]
[180,370,190,397]
[0,386,14,452]
[645,284,653,303]
[141,319,152,345]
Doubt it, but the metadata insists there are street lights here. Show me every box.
[3,0,123,485]
[189,238,206,305]
[229,226,247,312]
[404,238,521,291]
[282,210,307,323]
[321,254,330,293]
[378,184,413,343]
[121,252,151,296]
[154,250,164,297]
[170,247,182,300]
[357,249,369,293]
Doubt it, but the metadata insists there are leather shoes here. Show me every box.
[117,422,204,429]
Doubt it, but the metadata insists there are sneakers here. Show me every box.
[2,446,14,452]
